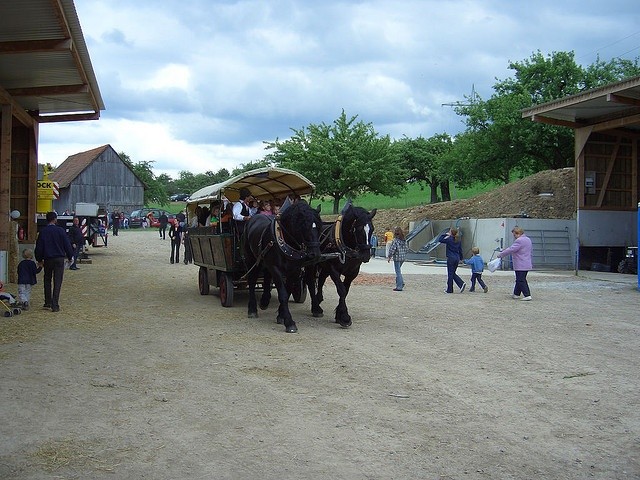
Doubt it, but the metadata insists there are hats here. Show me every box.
[238,187,252,200]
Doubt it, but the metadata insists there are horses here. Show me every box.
[305,204,377,328]
[242,202,322,333]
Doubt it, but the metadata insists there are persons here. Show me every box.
[119,211,125,230]
[169,218,182,264]
[158,212,168,240]
[63,210,69,215]
[176,210,185,222]
[497,227,533,300]
[388,227,407,291]
[384,228,394,261]
[233,187,280,239]
[0,280,19,306]
[70,210,75,216]
[370,232,378,260]
[146,212,155,228]
[181,223,192,264]
[462,246,488,293]
[189,200,233,226]
[69,218,84,270]
[17,248,44,306]
[111,209,120,236]
[34,212,76,312]
[437,227,466,294]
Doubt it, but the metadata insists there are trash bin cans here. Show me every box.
[625,246,638,275]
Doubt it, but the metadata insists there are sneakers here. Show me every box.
[520,296,532,301]
[394,287,402,290]
[52,308,60,311]
[484,286,488,294]
[511,294,520,299]
[23,302,29,310]
[460,283,466,293]
[43,303,52,307]
[444,289,452,294]
[469,287,476,291]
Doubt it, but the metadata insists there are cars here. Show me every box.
[129,207,177,229]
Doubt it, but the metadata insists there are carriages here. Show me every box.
[185,165,379,334]
